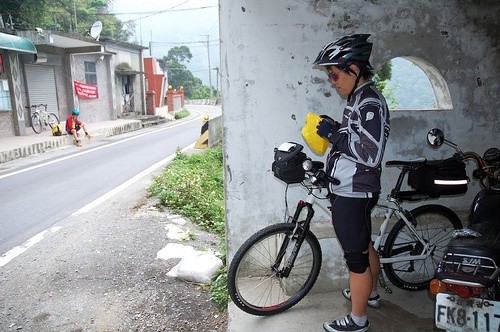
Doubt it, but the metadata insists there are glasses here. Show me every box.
[328,72,340,81]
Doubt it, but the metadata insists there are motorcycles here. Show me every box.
[425,127,500,332]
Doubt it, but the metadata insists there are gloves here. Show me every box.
[317,118,335,140]
[318,115,341,131]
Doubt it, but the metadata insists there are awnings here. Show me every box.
[119,70,146,75]
[0,32,38,54]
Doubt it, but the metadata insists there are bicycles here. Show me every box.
[25,103,59,134]
[224,142,464,318]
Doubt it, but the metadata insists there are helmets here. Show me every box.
[72,109,80,116]
[313,34,374,67]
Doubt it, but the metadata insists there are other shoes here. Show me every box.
[74,139,82,147]
[85,134,93,138]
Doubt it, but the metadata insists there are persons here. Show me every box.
[66,109,92,146]
[313,34,390,332]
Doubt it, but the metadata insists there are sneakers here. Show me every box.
[323,315,371,332]
[342,288,382,308]
